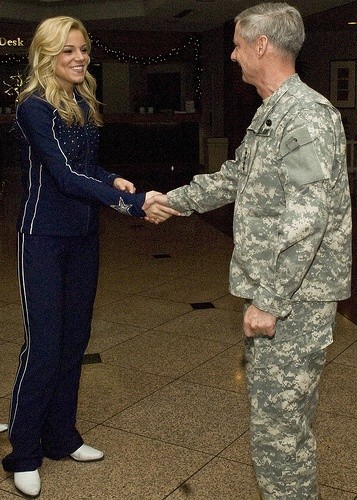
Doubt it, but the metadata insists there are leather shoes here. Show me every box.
[14,469,41,498]
[69,444,104,463]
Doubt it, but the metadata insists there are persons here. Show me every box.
[3,15,181,496]
[140,2,353,500]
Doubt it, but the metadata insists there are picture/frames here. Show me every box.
[329,60,356,109]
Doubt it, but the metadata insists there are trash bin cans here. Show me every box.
[205,136,229,175]
